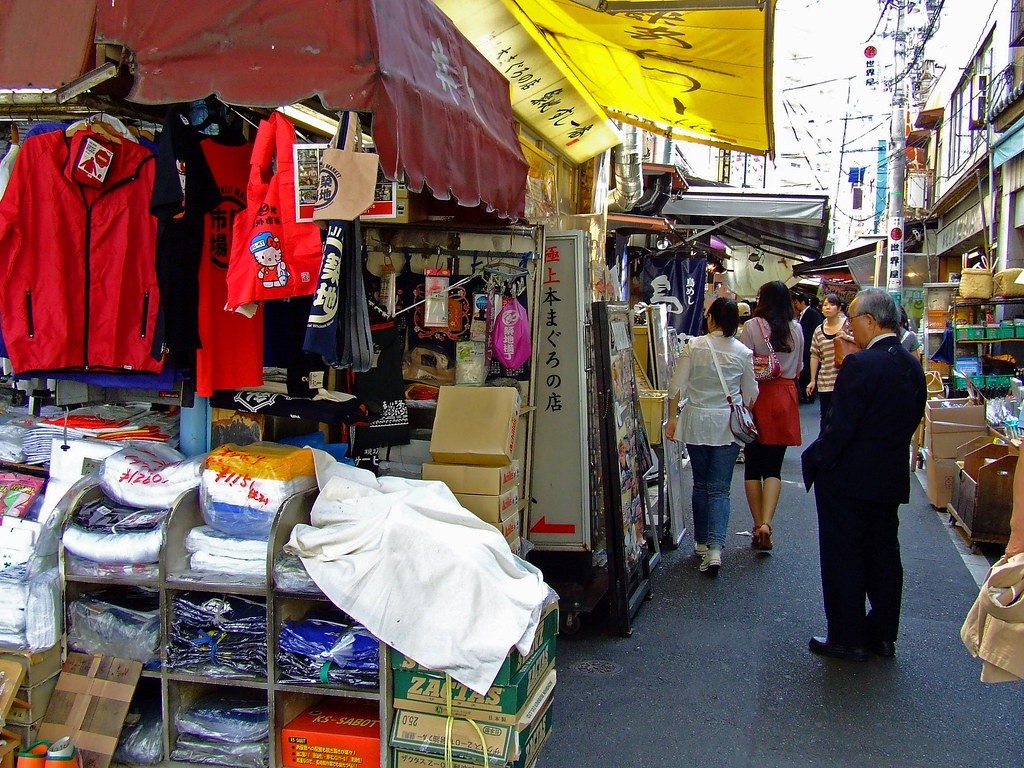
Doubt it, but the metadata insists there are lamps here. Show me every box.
[656,233,668,250]
[748,248,765,271]
[271,102,374,148]
[708,256,727,275]
[671,189,683,203]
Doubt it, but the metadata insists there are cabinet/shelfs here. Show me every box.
[951,292,1024,397]
[58,482,397,768]
[948,436,1019,552]
[921,283,960,385]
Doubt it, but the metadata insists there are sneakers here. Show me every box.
[700,549,721,572]
[693,543,710,555]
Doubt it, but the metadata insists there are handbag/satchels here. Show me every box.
[833,337,862,368]
[753,317,781,382]
[730,406,757,445]
[227,110,323,308]
[313,112,380,230]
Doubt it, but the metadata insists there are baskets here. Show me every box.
[925,370,944,394]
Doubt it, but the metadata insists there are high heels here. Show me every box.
[758,524,772,550]
[751,526,762,544]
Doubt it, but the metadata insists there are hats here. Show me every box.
[737,303,751,316]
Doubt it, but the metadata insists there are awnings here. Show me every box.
[662,187,831,260]
[505,0,775,160]
[95,0,530,223]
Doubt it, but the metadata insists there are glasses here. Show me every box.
[756,297,761,303]
[845,313,876,326]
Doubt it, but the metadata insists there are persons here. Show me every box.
[667,297,760,571]
[806,294,855,430]
[740,280,805,551]
[793,295,823,403]
[800,286,927,660]
[897,305,921,358]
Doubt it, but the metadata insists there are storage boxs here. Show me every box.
[925,309,1024,510]
[360,180,428,223]
[421,385,523,557]
[638,388,668,446]
[281,601,560,768]
[0,638,61,750]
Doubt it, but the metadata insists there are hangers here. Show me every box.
[6,104,246,146]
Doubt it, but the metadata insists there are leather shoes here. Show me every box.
[867,636,893,657]
[809,635,866,661]
